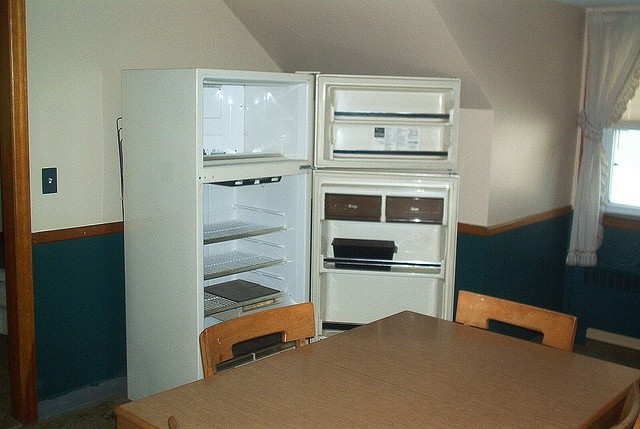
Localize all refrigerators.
[119,67,473,406]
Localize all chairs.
[454,290,578,351]
[199,302,316,379]
